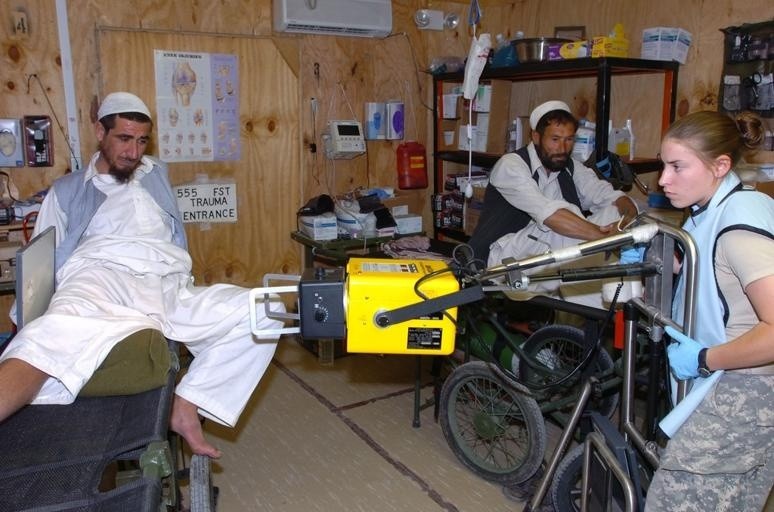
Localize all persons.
[0,90,289,459]
[641,109,774,511]
[466,99,642,303]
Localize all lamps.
[414,10,431,26]
[443,12,460,30]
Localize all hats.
[529,101,573,131]
[97,91,153,125]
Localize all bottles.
[624,118,635,161]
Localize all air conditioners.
[271,0,393,39]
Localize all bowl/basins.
[511,38,574,64]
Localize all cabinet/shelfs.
[432,55,679,340]
[717,19,774,200]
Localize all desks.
[290,229,473,427]
[0,221,36,291]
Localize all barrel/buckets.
[608,126,630,163]
[397,140,428,189]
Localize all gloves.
[664,325,705,381]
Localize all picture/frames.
[553,25,586,43]
